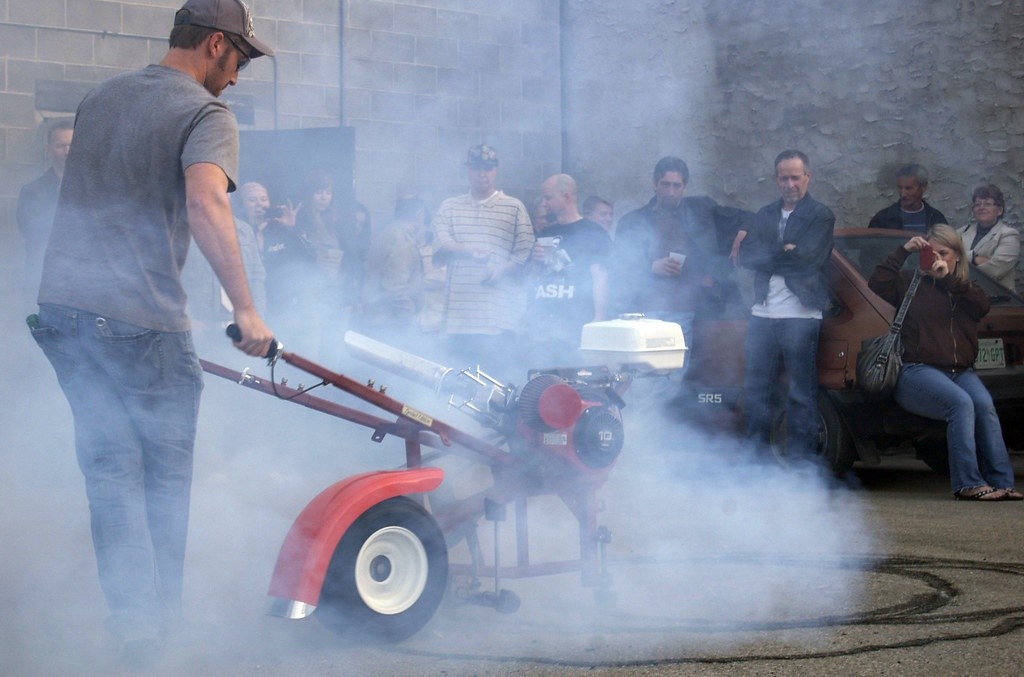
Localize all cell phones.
[920,246,933,271]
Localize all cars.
[680,228,1023,490]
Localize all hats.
[464,144,498,166]
[174,0,274,59]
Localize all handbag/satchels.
[855,333,905,400]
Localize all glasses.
[974,201,1002,207]
[470,164,493,171]
[211,31,251,71]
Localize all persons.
[583,196,614,232]
[522,174,614,323]
[182,145,535,351]
[957,184,1020,296]
[530,198,555,232]
[739,150,836,464]
[39,0,278,635]
[868,223,1024,502]
[16,121,73,263]
[859,164,948,278]
[614,156,757,414]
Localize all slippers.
[1005,488,1024,499]
[955,486,1008,500]
